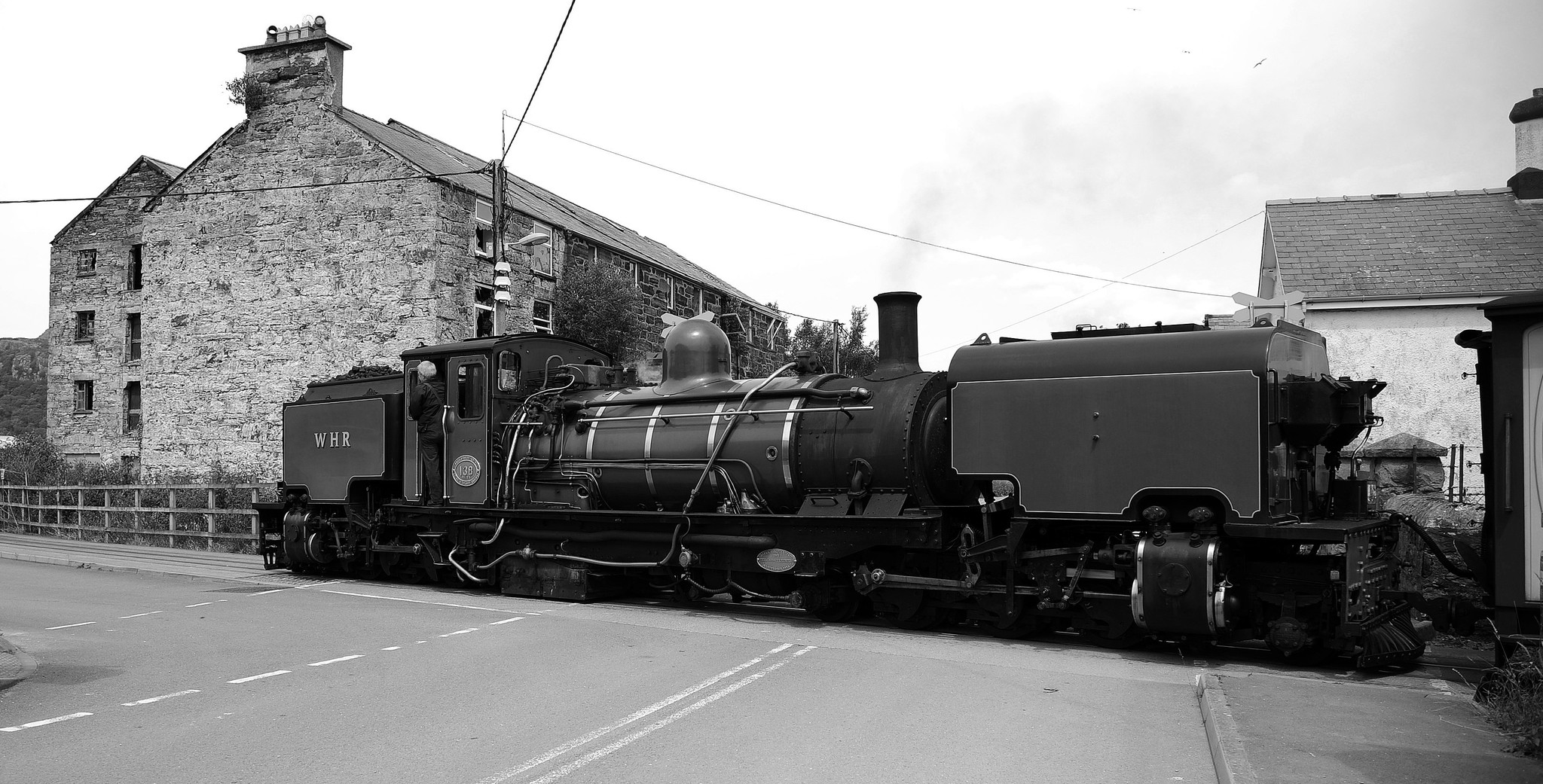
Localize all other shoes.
[426,499,444,507]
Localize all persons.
[409,360,446,507]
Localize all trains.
[248,291,1438,681]
